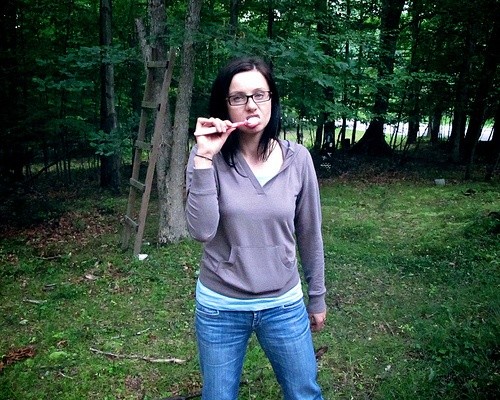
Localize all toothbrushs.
[194,117,261,136]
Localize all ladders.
[120,45,177,258]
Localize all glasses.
[225,91,272,106]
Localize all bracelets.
[196,154,213,163]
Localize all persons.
[185,58,327,400]
